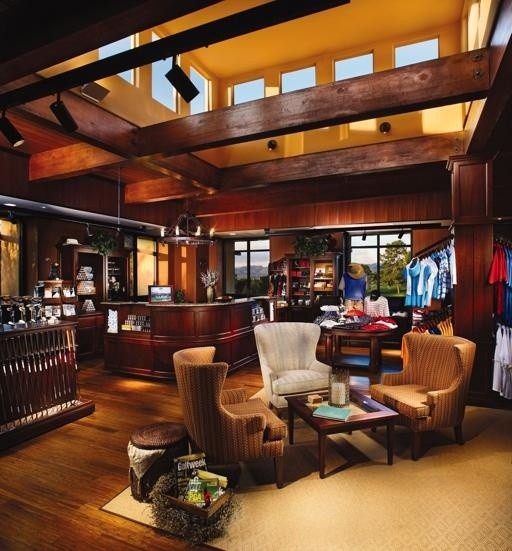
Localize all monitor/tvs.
[148,285,175,304]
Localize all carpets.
[100,388,512,551]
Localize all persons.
[362,290,390,318]
[337,270,369,313]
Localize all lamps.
[165,56,201,104]
[157,200,220,248]
[1,93,79,149]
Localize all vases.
[206,286,214,304]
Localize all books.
[312,405,352,422]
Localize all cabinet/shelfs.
[284,252,345,320]
[57,244,136,362]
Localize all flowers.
[200,269,222,288]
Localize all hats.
[346,262,364,279]
